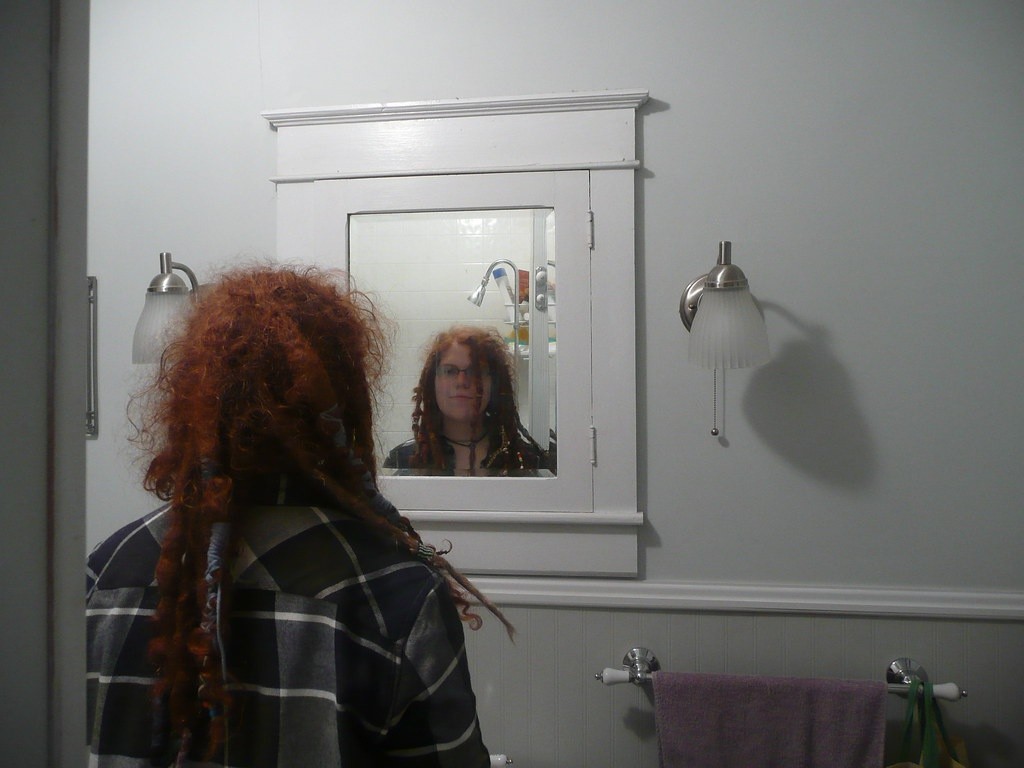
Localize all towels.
[650,670,887,768]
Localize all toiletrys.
[492,265,529,324]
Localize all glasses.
[436,363,489,379]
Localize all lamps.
[131,252,198,365]
[681,240,773,435]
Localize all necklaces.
[440,431,489,476]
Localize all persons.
[84,268,515,768]
[382,326,551,476]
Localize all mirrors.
[347,207,557,477]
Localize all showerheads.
[466,259,519,326]
